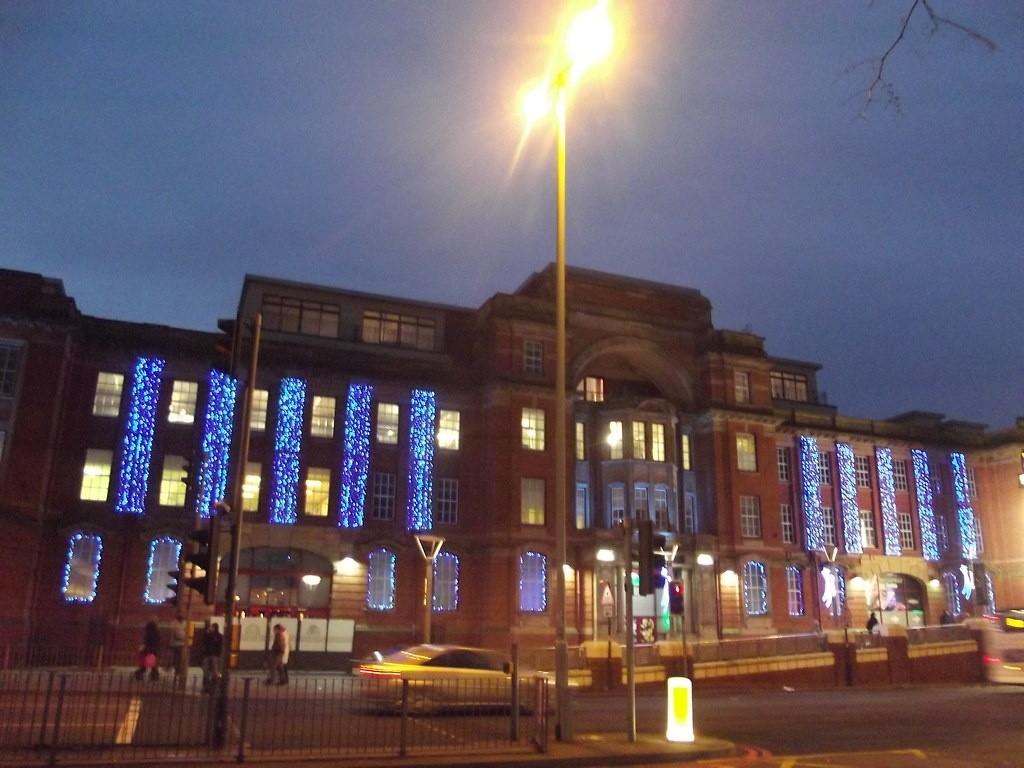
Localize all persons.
[866,613,878,634]
[134,622,161,680]
[169,613,185,676]
[264,624,289,685]
[940,609,970,624]
[200,624,224,693]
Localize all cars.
[351,643,578,716]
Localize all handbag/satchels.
[137,649,156,668]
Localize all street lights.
[516,8,625,741]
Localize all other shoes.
[263,679,274,684]
[275,680,287,686]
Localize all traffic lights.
[638,519,666,596]
[167,565,185,616]
[184,516,218,604]
[669,582,683,615]
[181,453,203,492]
[215,314,240,357]
